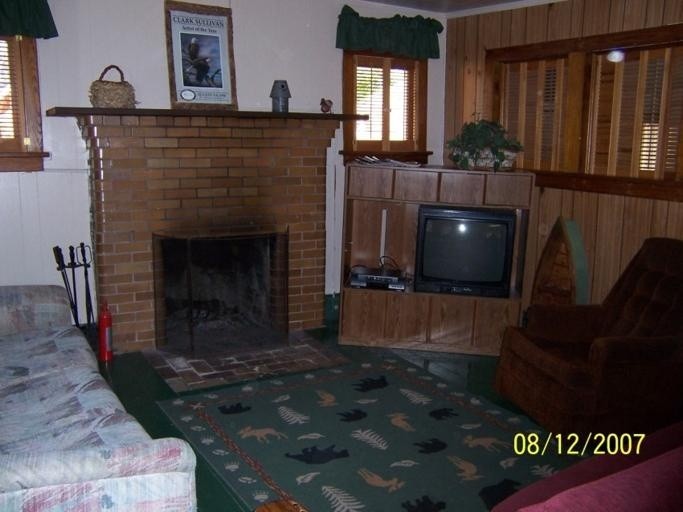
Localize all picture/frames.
[166,1,236,113]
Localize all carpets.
[157,353,579,511]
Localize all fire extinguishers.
[97,298,112,362]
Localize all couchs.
[0,284,197,512]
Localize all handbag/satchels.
[89,81,136,109]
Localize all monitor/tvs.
[413,204,516,299]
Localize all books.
[349,154,422,170]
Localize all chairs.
[491,236,681,450]
[487,422,683,512]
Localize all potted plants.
[446,119,524,171]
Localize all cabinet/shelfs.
[336,161,537,356]
